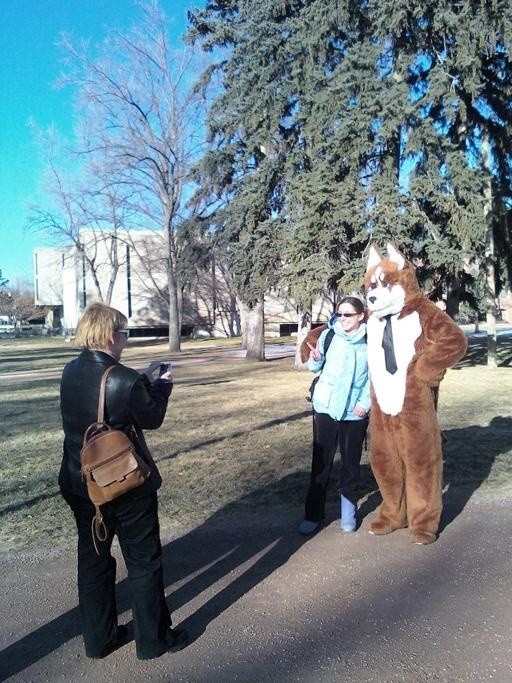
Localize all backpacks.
[78,362,153,527]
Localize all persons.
[298,295,371,537]
[57,301,190,660]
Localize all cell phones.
[159,364,169,377]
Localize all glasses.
[118,329,130,337]
[335,313,359,317]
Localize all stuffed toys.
[299,242,468,544]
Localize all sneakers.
[298,520,320,535]
